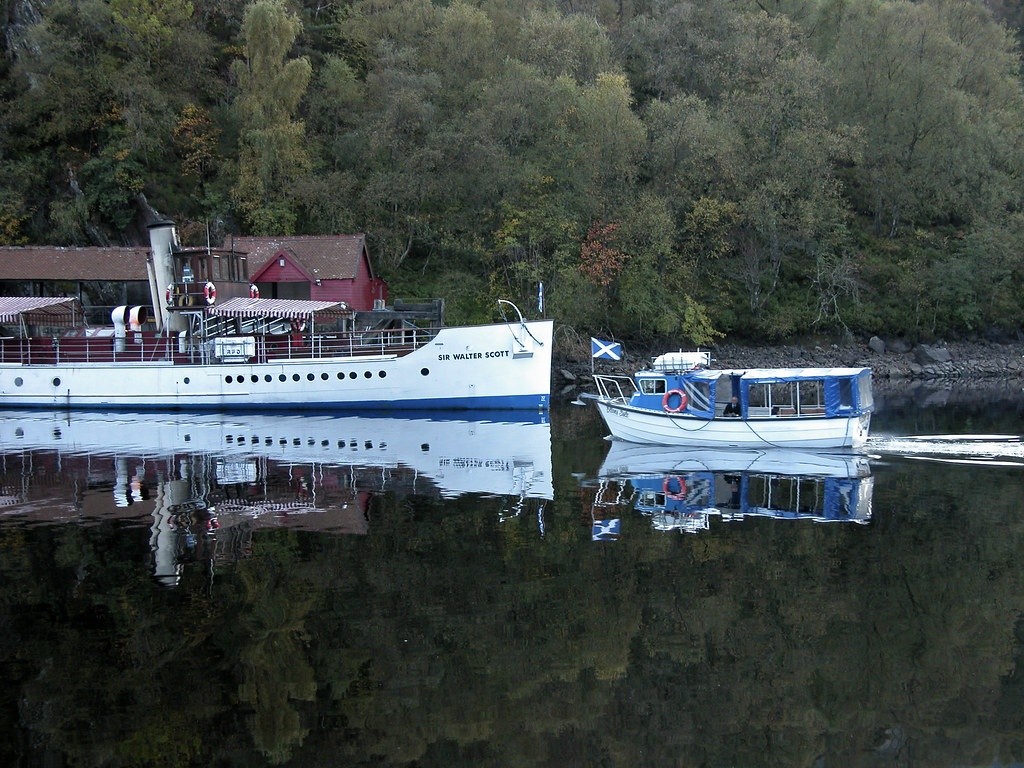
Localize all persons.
[723,397,742,417]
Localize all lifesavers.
[204,282,216,304]
[178,294,194,307]
[167,284,178,304]
[663,477,687,500]
[662,387,687,413]
[249,286,258,298]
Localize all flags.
[591,337,621,361]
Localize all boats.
[590,439,876,544]
[580,336,876,450]
[1,219,556,414]
[2,409,556,584]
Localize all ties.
[733,404,735,408]
